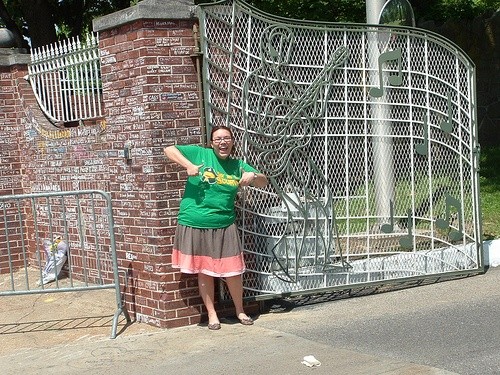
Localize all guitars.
[251,46,349,179]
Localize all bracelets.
[251,172,257,186]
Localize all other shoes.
[237,316,253,325]
[208,321,220,330]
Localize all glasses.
[211,136,234,143]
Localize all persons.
[162,125,269,329]
